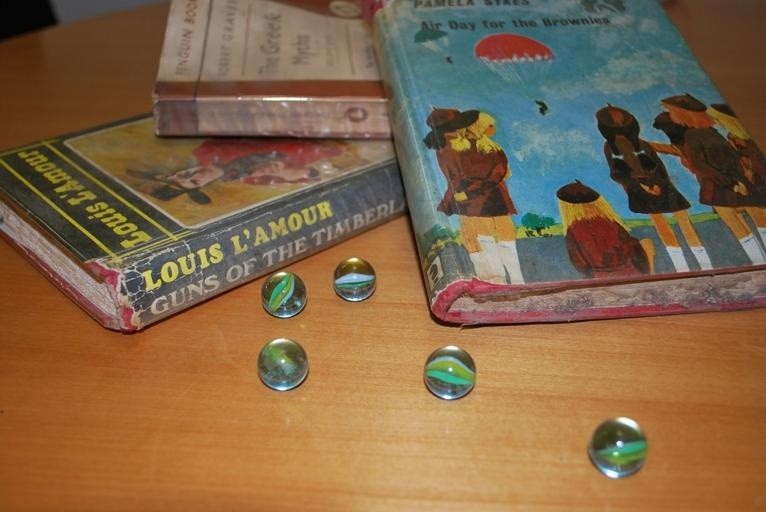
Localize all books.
[364,0,765,327]
[151,1,390,144]
[0,113,408,332]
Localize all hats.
[125,166,213,205]
[662,92,707,112]
[595,103,634,129]
[712,104,736,118]
[426,108,480,132]
[554,180,600,204]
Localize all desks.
[1,2,766,511]
